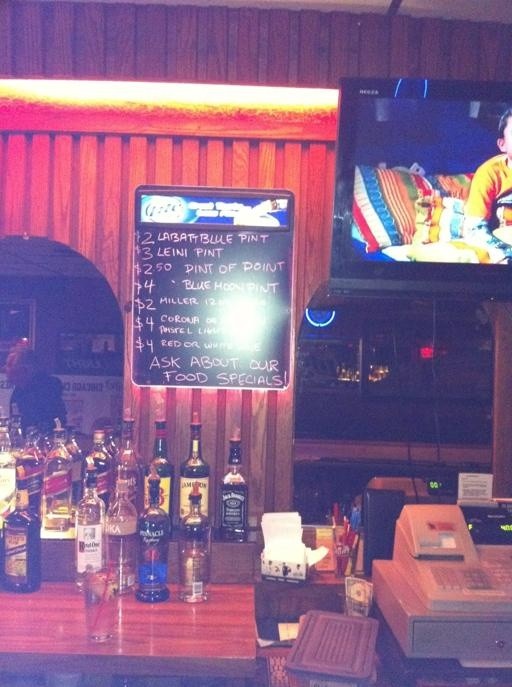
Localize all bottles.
[0,414,176,602]
[177,482,209,603]
[177,423,209,526]
[218,429,248,544]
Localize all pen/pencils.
[331,501,365,575]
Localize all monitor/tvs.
[327,75,512,301]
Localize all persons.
[9,350,67,442]
[460,107,512,265]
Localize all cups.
[83,569,115,642]
[332,527,357,580]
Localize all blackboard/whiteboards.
[131,185,295,390]
